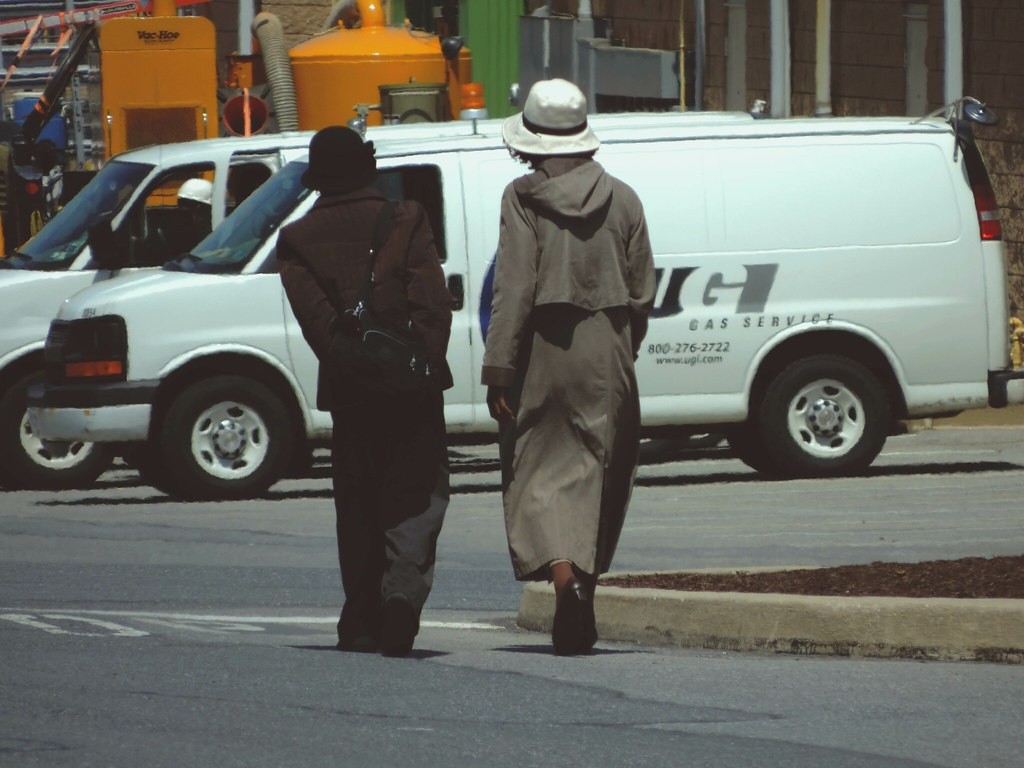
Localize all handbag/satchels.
[354,200,432,414]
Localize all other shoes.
[337,618,372,647]
[552,577,598,650]
[381,601,416,653]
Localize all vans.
[0,109,758,497]
[24,103,1014,480]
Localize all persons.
[278,125,453,657]
[481,79,657,654]
[157,179,212,260]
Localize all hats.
[299,125,376,194]
[502,77,600,154]
[177,177,214,205]
